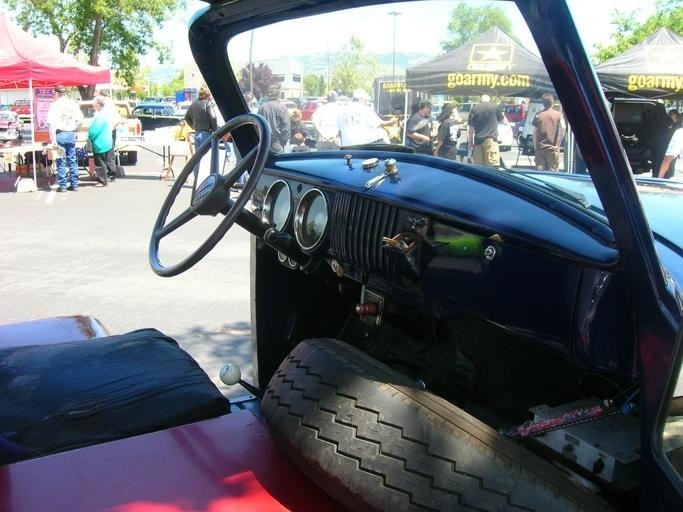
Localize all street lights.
[388,12,403,81]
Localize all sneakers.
[67,186,78,191]
[57,188,65,192]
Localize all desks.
[0,140,225,190]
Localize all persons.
[231,87,254,183]
[432,102,461,159]
[87,95,116,187]
[46,84,84,192]
[667,109,681,126]
[258,85,399,154]
[466,94,505,168]
[405,98,435,154]
[529,93,567,172]
[658,127,683,183]
[93,88,121,146]
[184,85,218,171]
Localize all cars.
[457,102,515,153]
[133,95,192,125]
[499,104,526,123]
[258,96,269,107]
[298,100,322,125]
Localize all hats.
[54,85,65,93]
[419,99,432,107]
[354,89,370,100]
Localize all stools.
[0,325,228,469]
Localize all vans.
[608,98,664,173]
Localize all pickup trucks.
[77,99,144,163]
